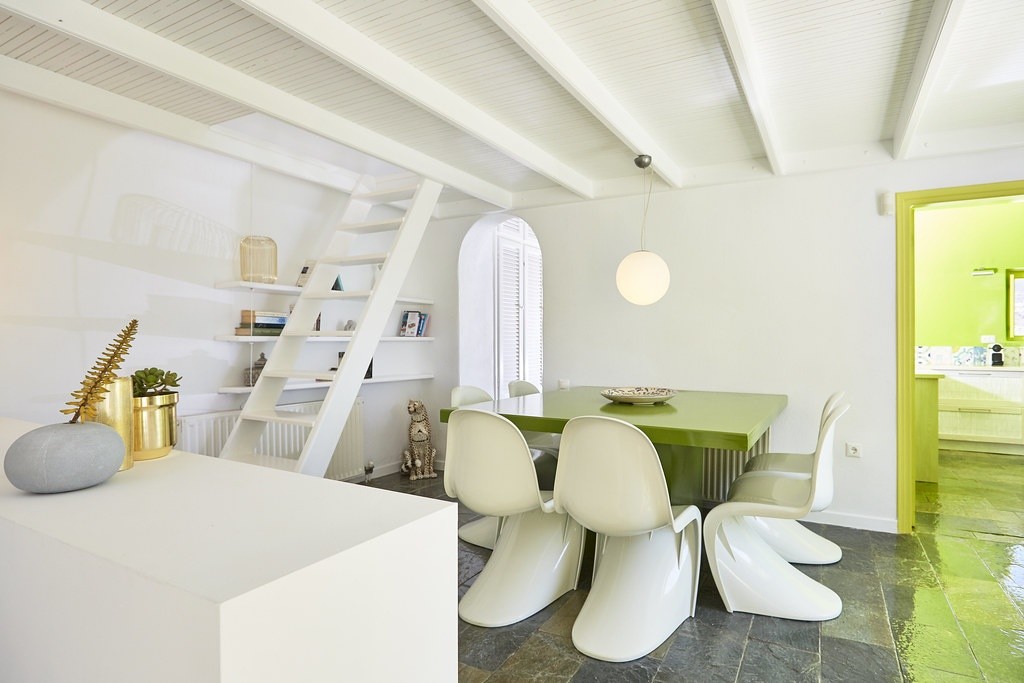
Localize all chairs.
[553,415,702,663]
[451,385,548,546]
[706,403,857,623]
[737,380,848,565]
[506,380,566,462]
[443,407,583,627]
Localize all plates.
[600,385,679,405]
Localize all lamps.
[617,146,675,307]
[172,399,369,481]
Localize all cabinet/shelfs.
[217,281,434,397]
[0,411,458,683]
[922,365,1024,456]
[914,365,945,483]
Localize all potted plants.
[130,366,181,461]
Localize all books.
[297,267,312,287]
[235,310,289,335]
[400,311,429,337]
[332,275,343,290]
[313,315,320,331]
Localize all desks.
[441,385,790,559]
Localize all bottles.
[240,235,278,284]
